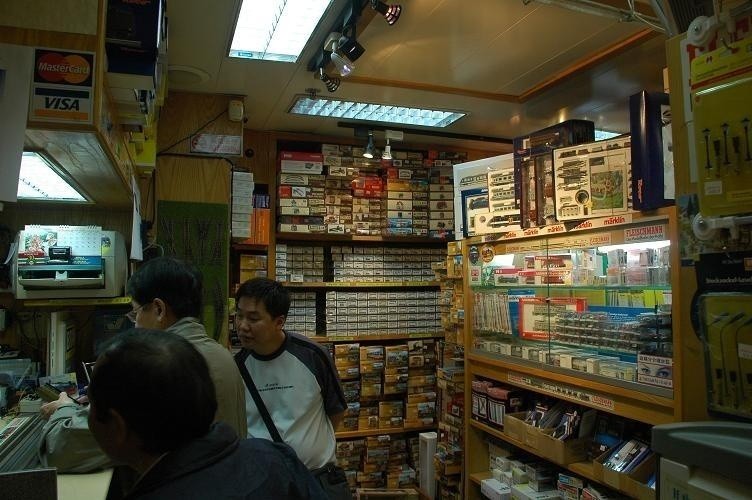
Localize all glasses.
[124,304,154,323]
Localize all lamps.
[307,0,401,92]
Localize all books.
[0,414,46,476]
[475,290,535,338]
[106,17,167,178]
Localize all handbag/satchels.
[307,462,351,500]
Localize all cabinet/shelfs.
[465,205,683,500]
[269,132,464,500]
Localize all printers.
[17,229,128,299]
